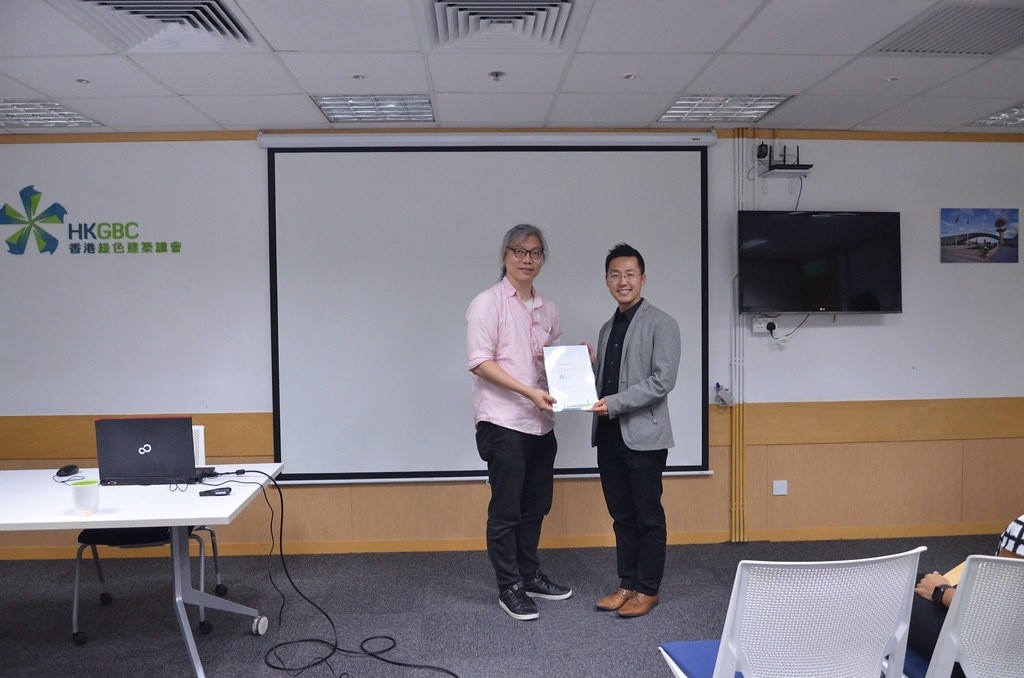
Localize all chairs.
[903,555,1024,678]
[71,425,226,646]
[659,543,928,678]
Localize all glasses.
[507,246,543,260]
[607,272,642,282]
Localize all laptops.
[95,417,215,486]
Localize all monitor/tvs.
[737,210,903,316]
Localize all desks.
[0,461,283,678]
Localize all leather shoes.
[596,587,634,610]
[618,592,658,617]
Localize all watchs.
[932,585,951,603]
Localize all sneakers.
[498,580,539,620]
[521,569,572,600]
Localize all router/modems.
[768,145,813,170]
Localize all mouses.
[56,464,79,477]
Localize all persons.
[579,243,681,617]
[907,514,1024,678]
[467,223,573,620]
